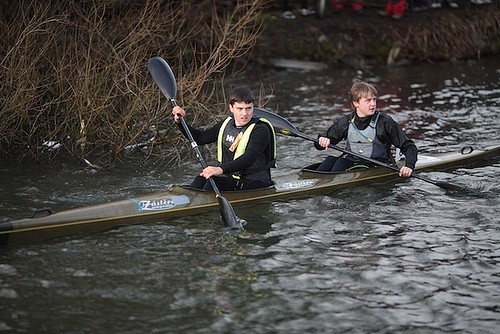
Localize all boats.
[0,141,500,246]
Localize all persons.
[172,87,277,191]
[314,82,418,177]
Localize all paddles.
[252,108,475,191]
[147,56,238,226]
